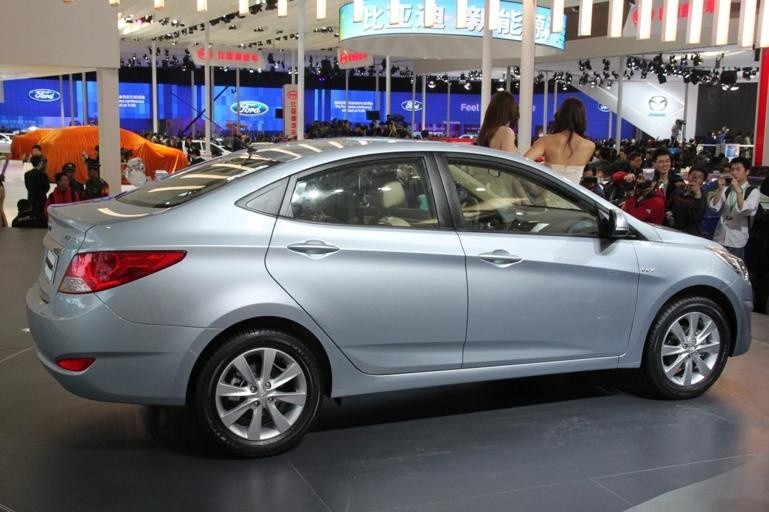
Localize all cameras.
[725,176,734,187]
[624,181,635,191]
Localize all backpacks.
[725,185,769,252]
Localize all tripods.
[671,130,682,148]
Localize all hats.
[63,159,100,172]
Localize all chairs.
[376,181,412,227]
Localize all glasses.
[630,159,704,179]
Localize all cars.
[24,135,755,460]
[457,134,479,146]
[0,132,19,155]
[158,137,276,174]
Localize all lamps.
[109,0,769,94]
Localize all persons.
[476,91,761,261]
[307,114,412,139]
[0,145,109,227]
[144,129,281,166]
